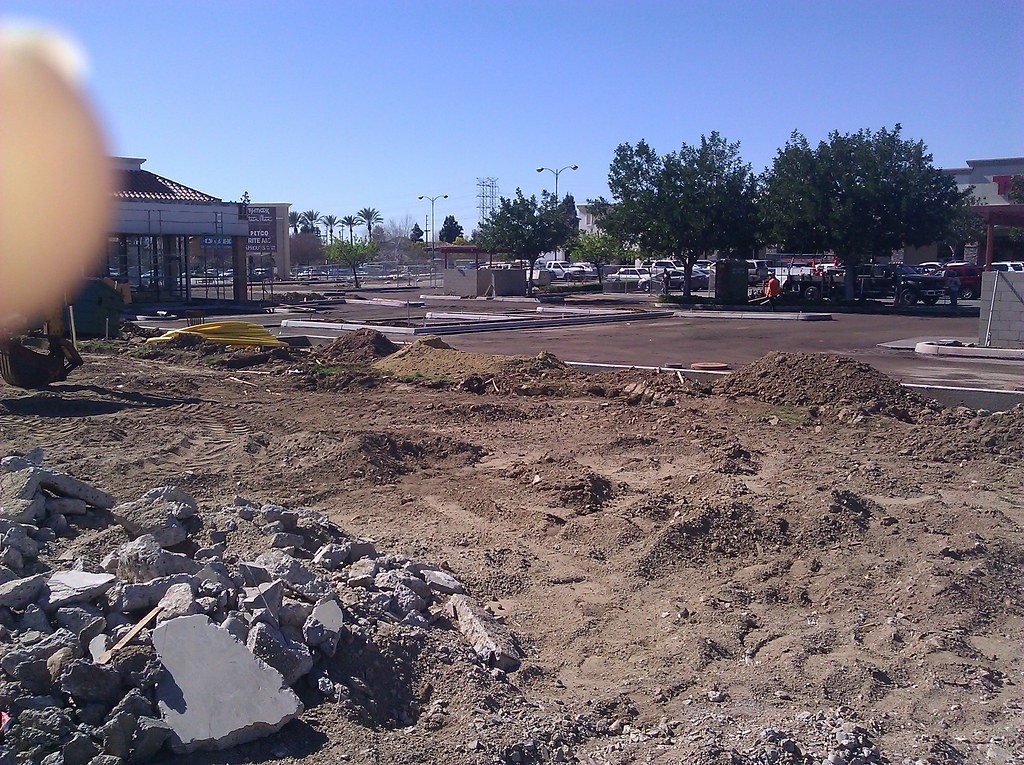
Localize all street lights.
[417,193,449,265]
[535,164,579,261]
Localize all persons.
[660,268,672,294]
[947,271,961,305]
[766,272,780,311]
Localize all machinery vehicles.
[0,275,132,390]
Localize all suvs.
[855,262,947,308]
[928,263,983,300]
[982,261,1024,271]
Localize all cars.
[605,258,847,304]
[110,265,323,280]
[463,261,597,282]
